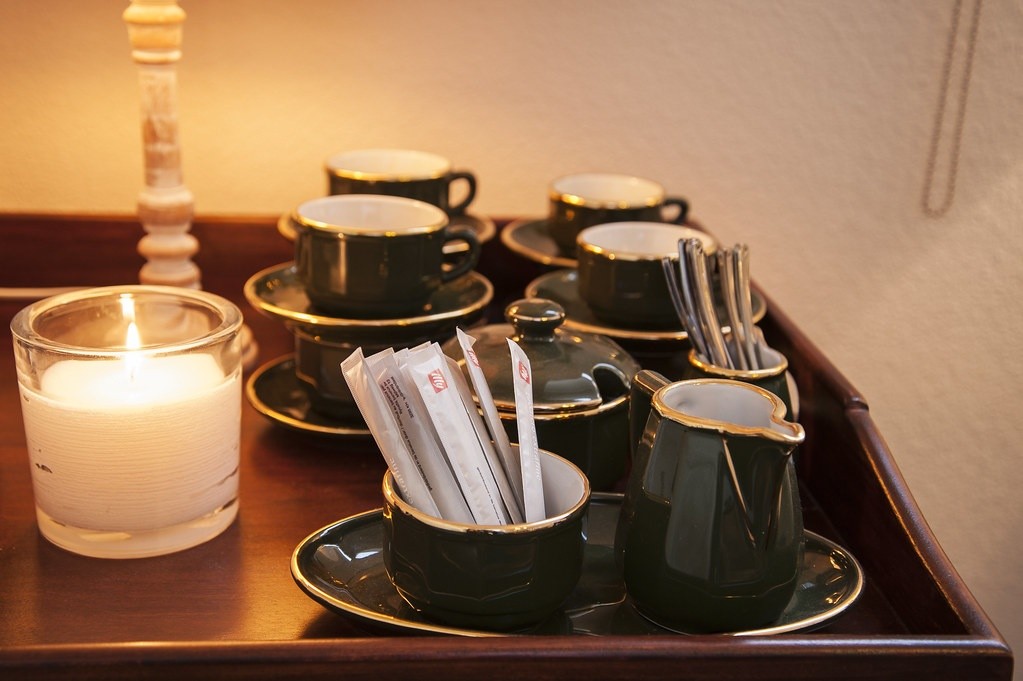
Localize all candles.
[42,320,227,404]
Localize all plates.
[287,496,863,638]
[278,208,496,255]
[243,260,494,336]
[245,348,368,436]
[528,268,767,345]
[500,213,714,267]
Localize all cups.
[294,192,478,315]
[686,341,794,423]
[9,284,244,558]
[324,148,478,224]
[291,328,364,406]
[381,442,592,628]
[548,172,690,257]
[575,222,721,325]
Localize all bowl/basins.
[437,296,644,495]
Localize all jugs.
[613,370,808,633]
